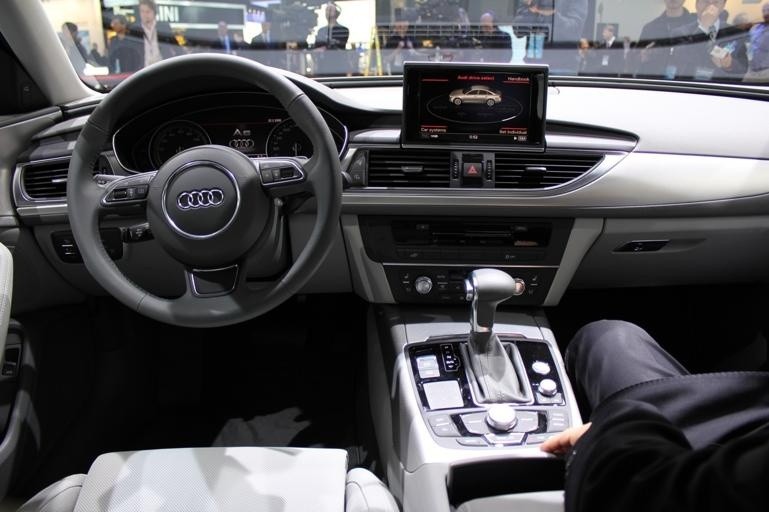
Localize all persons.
[210,21,239,57]
[539,319,769,512]
[59,0,186,74]
[250,20,280,68]
[384,18,419,74]
[480,0,769,84]
[314,2,349,76]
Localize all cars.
[449,85,502,107]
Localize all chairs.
[9,446,401,512]
